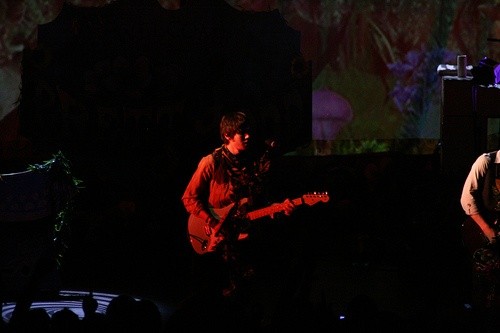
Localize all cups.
[457,55,467,77]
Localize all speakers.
[441,76,488,191]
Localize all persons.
[179,110,300,300]
[458,146,500,304]
[0,258,499,333]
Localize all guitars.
[462,209,500,252]
[187,188,332,254]
